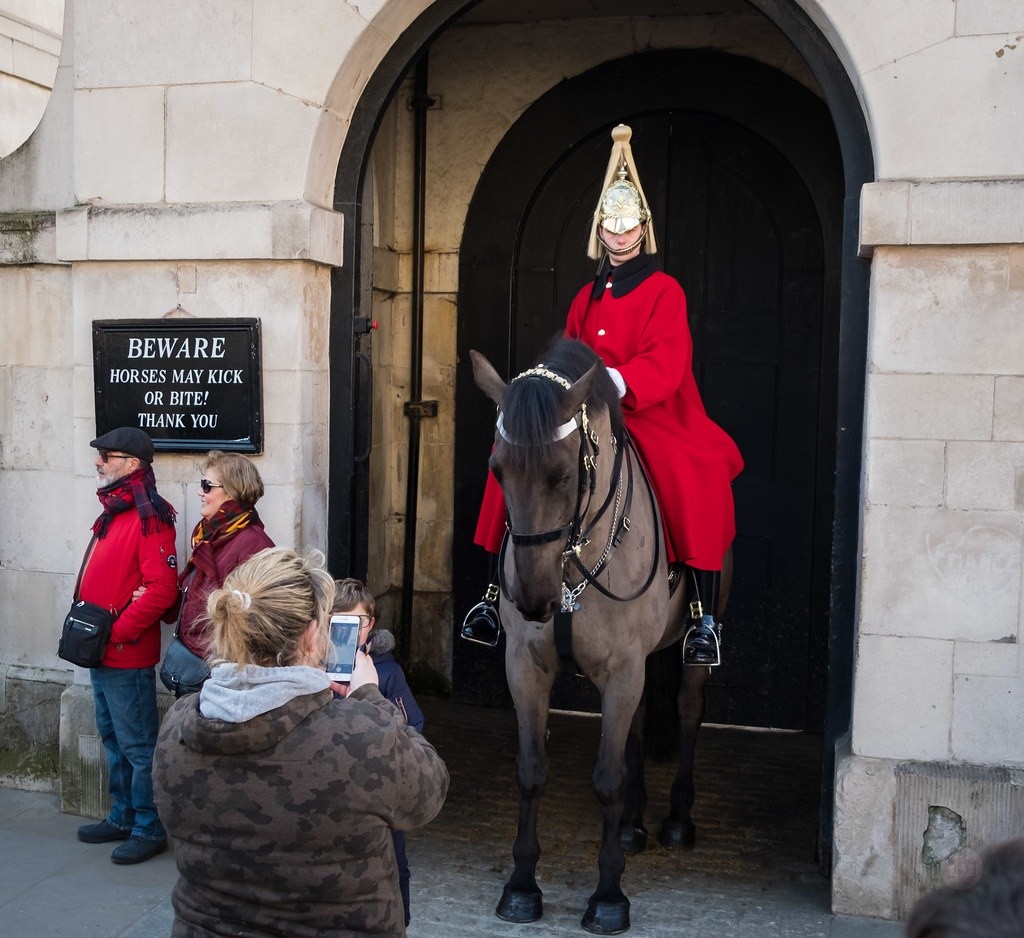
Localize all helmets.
[586,124,657,259]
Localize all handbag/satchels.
[160,639,210,698]
[59,600,113,668]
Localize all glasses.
[357,612,372,629]
[200,479,223,494]
[98,450,135,462]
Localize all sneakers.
[78,819,130,844]
[111,834,169,865]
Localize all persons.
[462,124,743,664]
[903,837,1024,938]
[73,427,179,865]
[329,578,424,928]
[131,451,276,699]
[151,546,450,938]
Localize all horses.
[465,333,735,931]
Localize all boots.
[684,565,720,664]
[462,553,502,645]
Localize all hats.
[89,427,154,463]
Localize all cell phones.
[323,616,362,683]
